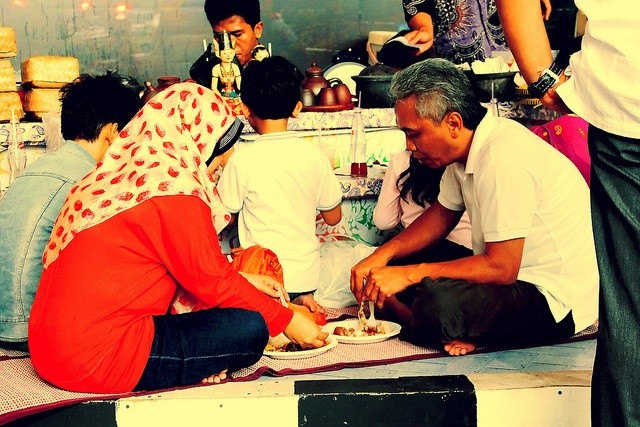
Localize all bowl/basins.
[473,71,520,102]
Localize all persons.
[529,33,591,189]
[250,42,272,61]
[29,81,330,393]
[495,0,640,426]
[0,68,139,352]
[373,149,473,249]
[204,0,264,69]
[216,55,343,317]
[402,0,552,65]
[211,29,242,105]
[350,57,600,355]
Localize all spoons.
[358,278,369,330]
[366,301,379,334]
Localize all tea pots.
[144,77,197,110]
[299,63,341,103]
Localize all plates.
[323,61,368,101]
[323,318,401,345]
[263,332,338,360]
[301,106,355,112]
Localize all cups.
[320,88,336,106]
[42,114,62,155]
[334,84,351,105]
[302,89,316,107]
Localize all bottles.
[6,119,27,187]
[352,108,367,178]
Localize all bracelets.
[527,61,563,99]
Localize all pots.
[351,62,402,108]
